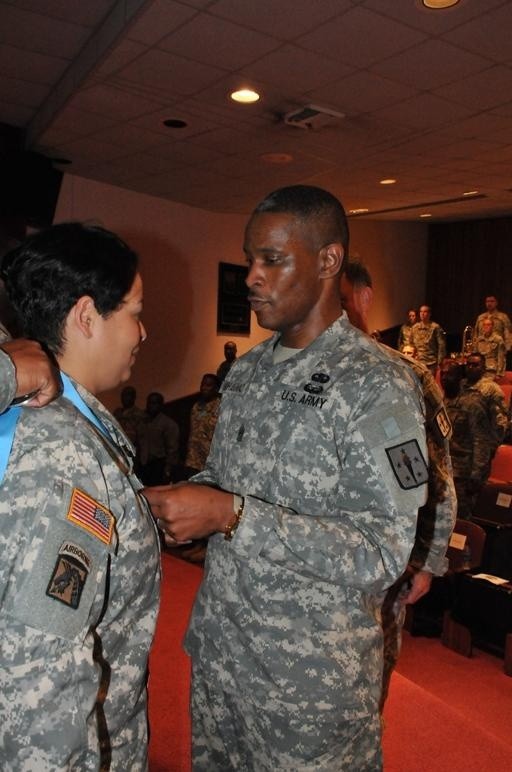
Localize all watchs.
[225,496,244,543]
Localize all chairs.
[406,355,512,677]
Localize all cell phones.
[11,390,40,405]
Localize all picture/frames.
[217,262,249,337]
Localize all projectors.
[281,103,345,132]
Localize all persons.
[113,294,511,623]
[138,185,432,770]
[334,261,458,737]
[0,221,164,771]
[0,305,60,413]
